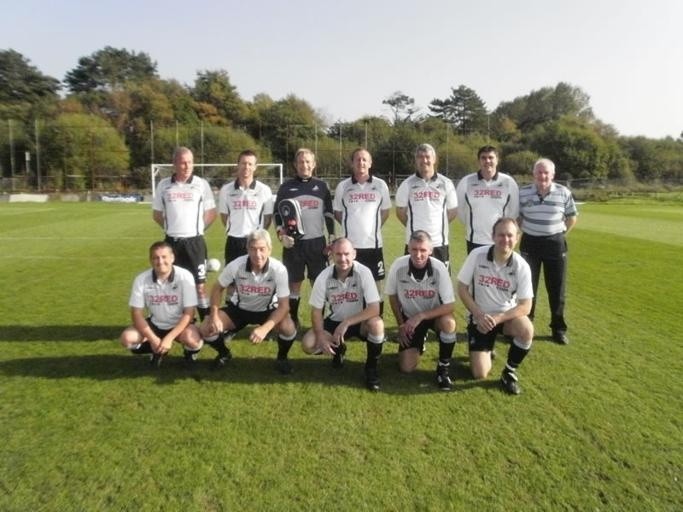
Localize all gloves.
[279,233,294,249]
[323,235,335,264]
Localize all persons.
[504,158,578,345]
[333,148,392,319]
[302,237,385,390]
[120,241,203,368]
[457,217,534,394]
[385,230,457,391]
[273,148,335,326]
[219,151,275,342]
[152,145,218,320]
[200,230,298,373]
[396,143,458,276]
[456,146,520,254]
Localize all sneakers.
[501,374,519,396]
[433,372,453,390]
[277,359,291,372]
[212,353,232,367]
[182,356,197,367]
[554,332,568,344]
[150,353,163,366]
[332,343,347,366]
[367,372,382,390]
